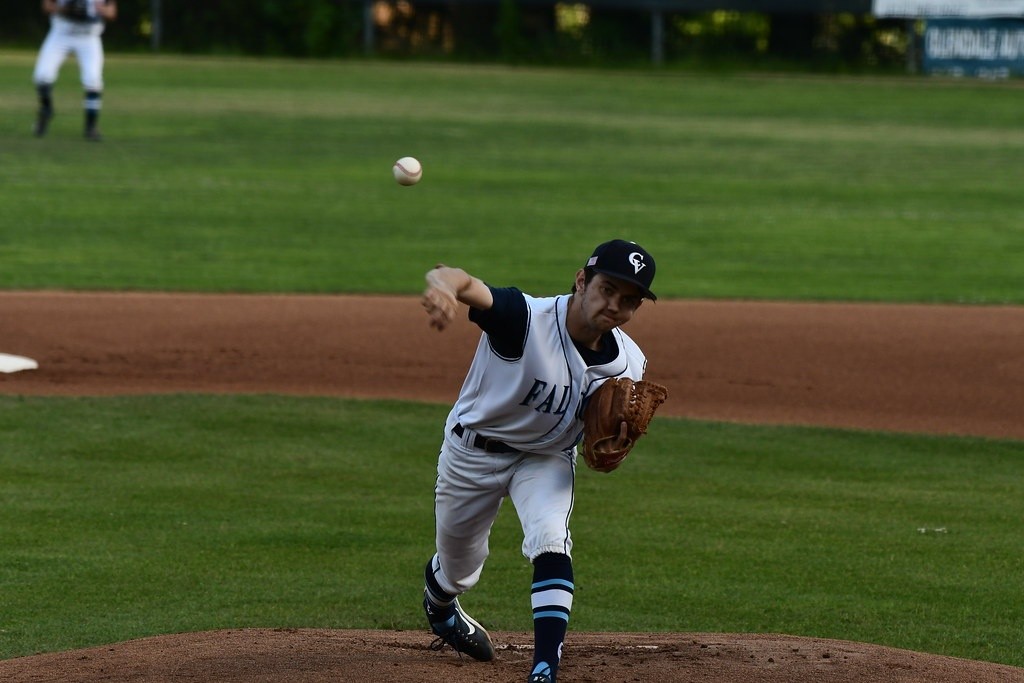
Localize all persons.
[31,0,118,140]
[421,240,669,683]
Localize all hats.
[586,239,658,300]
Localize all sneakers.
[423,587,495,662]
[527,666,556,683]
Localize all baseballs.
[393,154,423,186]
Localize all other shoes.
[85,130,100,141]
[33,111,48,137]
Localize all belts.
[452,423,522,453]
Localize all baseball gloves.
[582,373,671,474]
